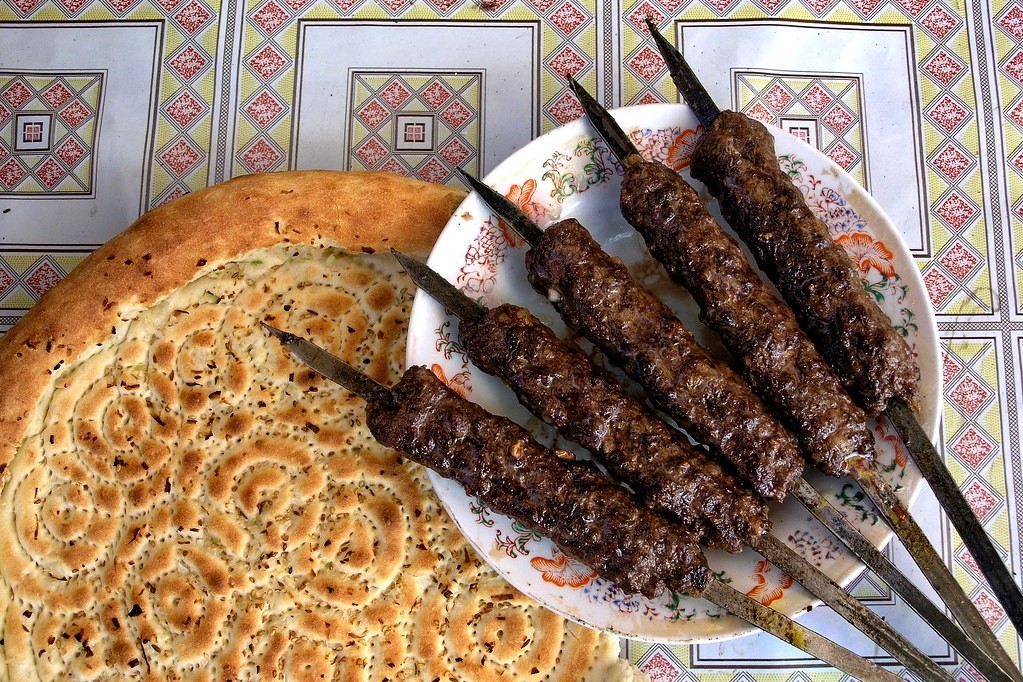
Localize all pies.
[0,169,647,682]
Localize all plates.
[403,99,946,643]
